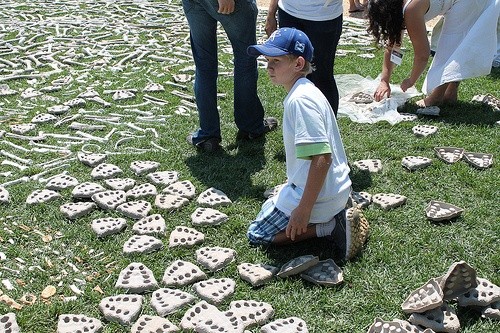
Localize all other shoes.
[347,202,370,248]
[186,133,221,146]
[237,134,265,145]
[349,8,364,12]
[397,100,430,114]
[330,207,362,262]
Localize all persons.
[367,0,500,112]
[182,0,278,151]
[247,28,371,261]
[263,0,357,118]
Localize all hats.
[247,27,314,63]
